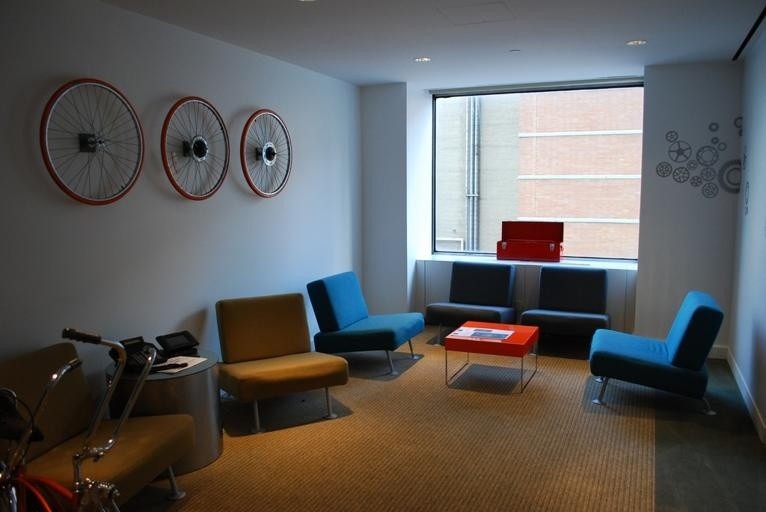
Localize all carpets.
[119,325,657,512]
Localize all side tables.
[105,349,223,478]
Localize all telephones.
[109,336,167,373]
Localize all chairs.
[426,261,517,346]
[0,343,197,512]
[589,290,724,416]
[215,293,349,431]
[306,271,426,377]
[520,265,611,361]
